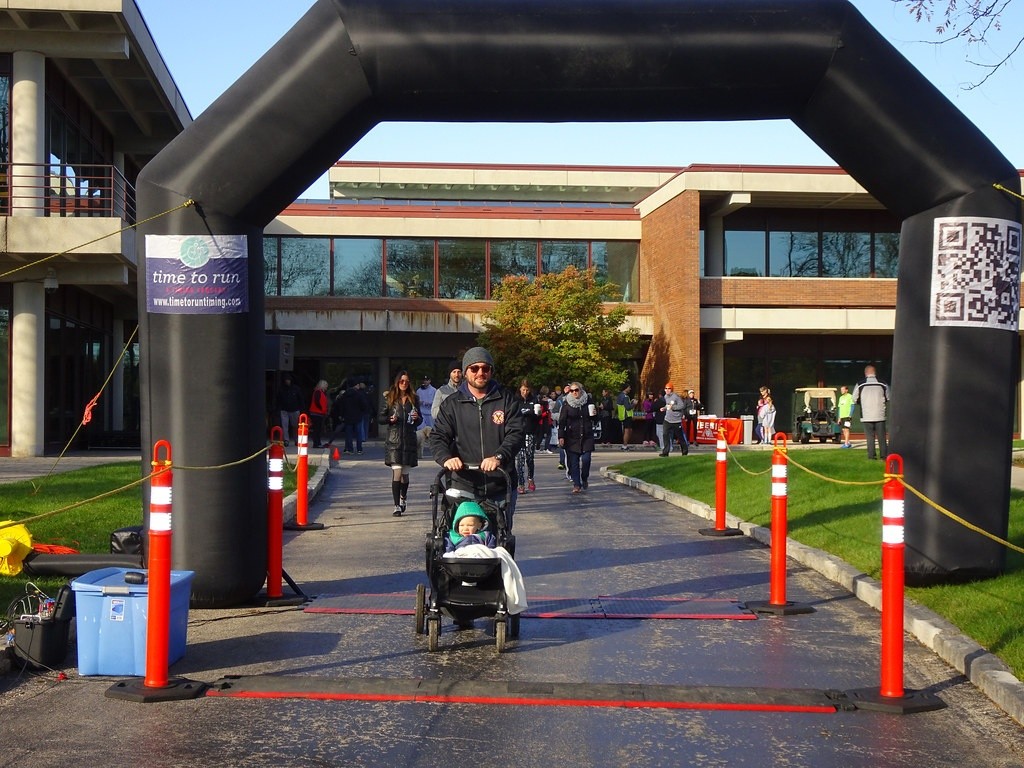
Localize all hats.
[462,347,495,377]
[621,381,630,390]
[424,375,431,381]
[688,390,694,394]
[555,386,561,391]
[646,391,655,395]
[665,382,673,392]
[448,361,462,379]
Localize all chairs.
[816,410,828,422]
[0,173,9,212]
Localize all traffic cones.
[333,448,340,459]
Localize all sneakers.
[358,446,364,454]
[543,449,553,454]
[527,477,535,491]
[519,484,524,494]
[535,450,543,454]
[399,491,407,511]
[583,480,587,489]
[841,443,851,449]
[558,463,566,469]
[572,484,581,493]
[342,449,354,455]
[392,504,401,517]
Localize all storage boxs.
[71,567,195,678]
[14,585,75,671]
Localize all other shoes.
[643,441,655,446]
[621,445,633,452]
[659,452,668,457]
[452,616,474,629]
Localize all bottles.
[407,407,416,424]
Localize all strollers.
[413,463,521,652]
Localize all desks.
[633,416,646,420]
[682,418,743,445]
[591,418,657,444]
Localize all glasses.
[570,389,579,393]
[399,380,409,385]
[468,365,492,373]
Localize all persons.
[378,370,424,517]
[444,502,496,553]
[279,362,465,454]
[837,385,854,449]
[755,385,777,444]
[430,347,526,626]
[852,365,890,460]
[515,380,701,493]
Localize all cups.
[588,403,595,416]
[534,404,540,414]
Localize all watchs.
[495,453,505,461]
